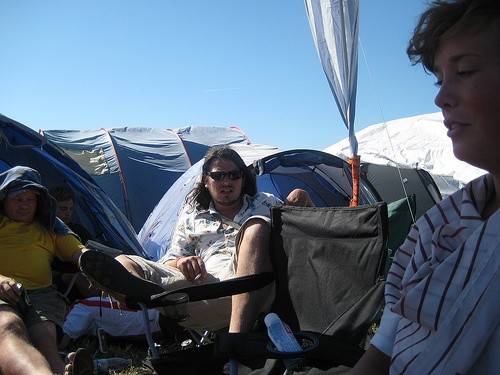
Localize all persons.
[344,0,500,375]
[78,147,316,355]
[0,165,110,375]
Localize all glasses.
[207,171,242,181]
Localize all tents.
[0,113,466,351]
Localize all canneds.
[180,339,195,349]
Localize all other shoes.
[79,250,165,308]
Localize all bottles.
[264,312,304,368]
[93,357,132,375]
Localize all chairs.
[141,194,418,375]
[51,258,84,294]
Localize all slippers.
[63,348,93,375]
